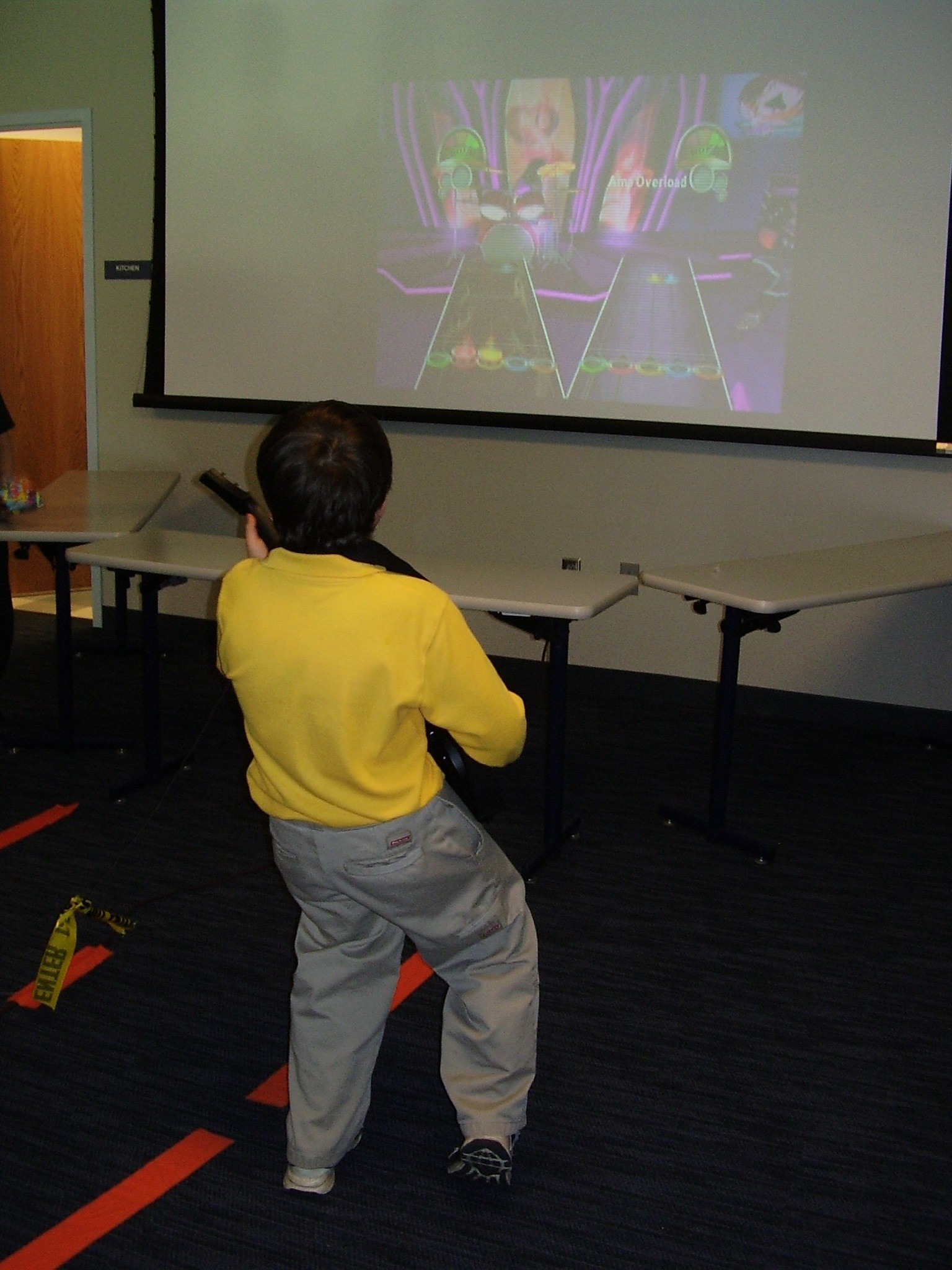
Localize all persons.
[0,393,15,727]
[215,399,541,1195]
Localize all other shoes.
[284,1166,338,1194]
[447,1137,516,1186]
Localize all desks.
[65,528,638,886]
[638,529,952,865]
[0,469,180,756]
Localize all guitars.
[192,476,469,785]
